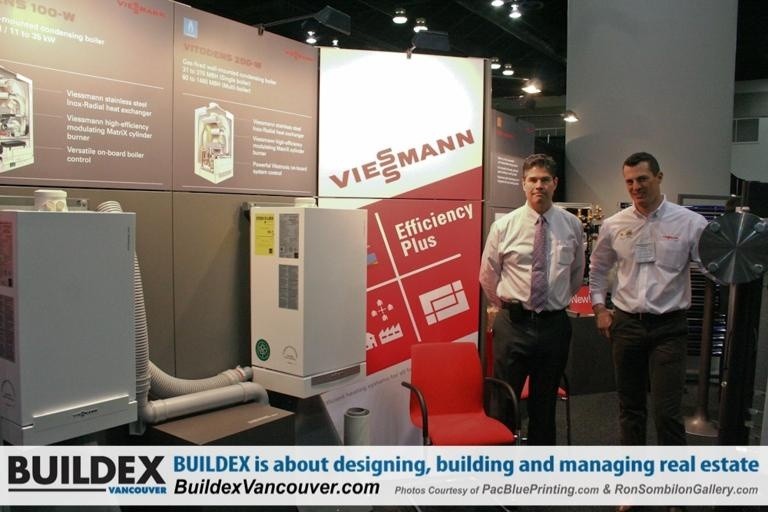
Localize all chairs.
[402,341,521,446]
[522,374,572,446]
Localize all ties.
[531,217,548,314]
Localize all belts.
[624,313,661,321]
[524,310,557,319]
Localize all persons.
[588,152,730,512]
[477,153,586,444]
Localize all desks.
[151,402,294,443]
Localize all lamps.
[392,1,429,35]
[489,51,514,76]
[520,77,542,94]
[491,0,521,19]
[306,26,339,46]
[562,112,579,122]
